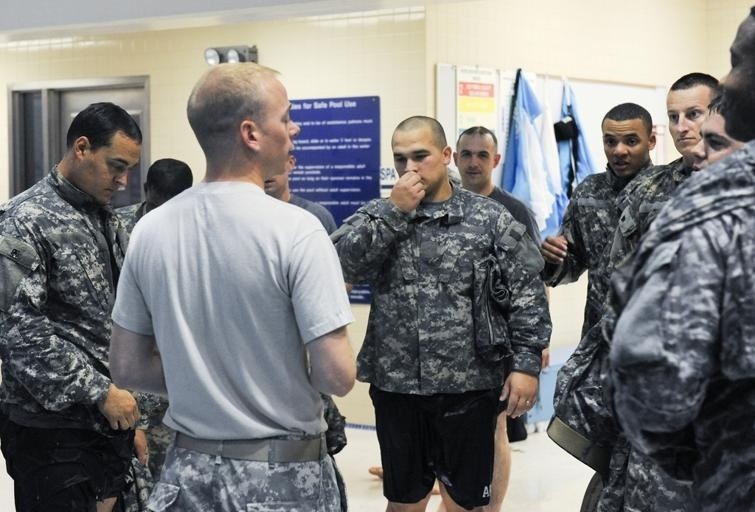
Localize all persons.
[369,467,440,495]
[112,158,193,512]
[264,150,352,297]
[329,116,553,512]
[108,62,357,512]
[0,102,149,512]
[540,4,755,510]
[436,126,549,512]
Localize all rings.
[526,400,532,406]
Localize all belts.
[548,416,610,472]
[175,433,320,463]
